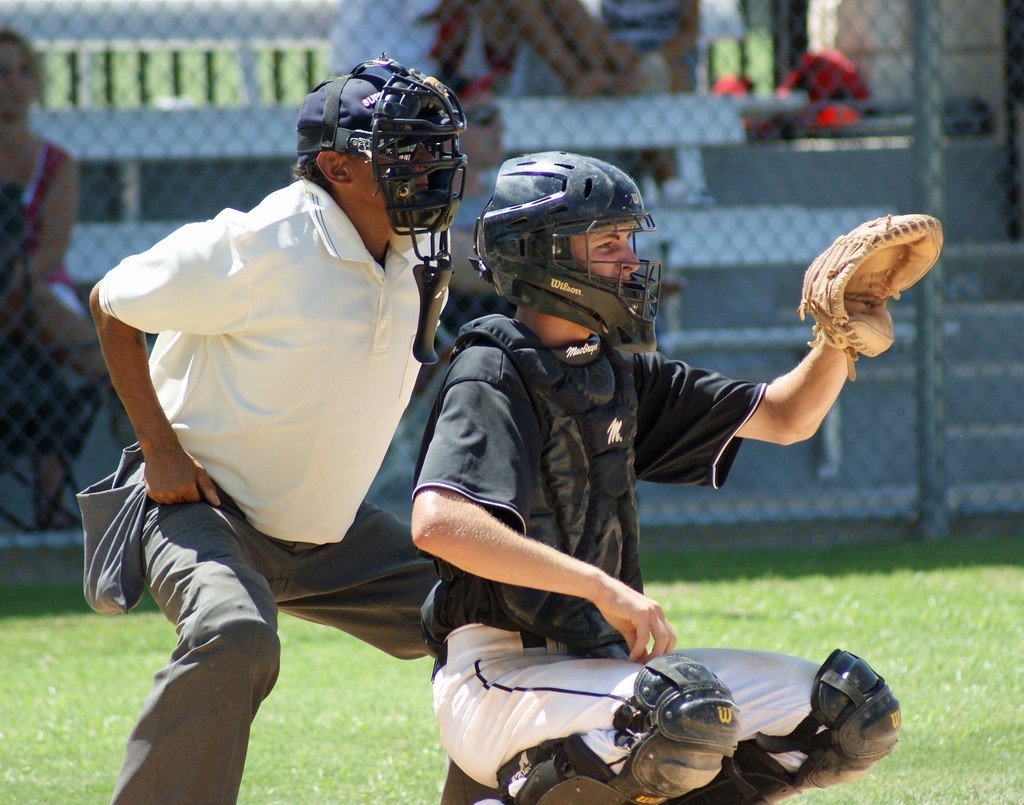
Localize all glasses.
[466,106,501,125]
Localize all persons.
[0,28,107,529]
[326,0,701,204]
[90,65,469,805]
[412,146,943,805]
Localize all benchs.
[28,90,898,485]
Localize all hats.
[296,73,458,165]
[431,63,505,102]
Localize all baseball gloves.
[795,212,944,385]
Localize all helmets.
[468,149,663,353]
